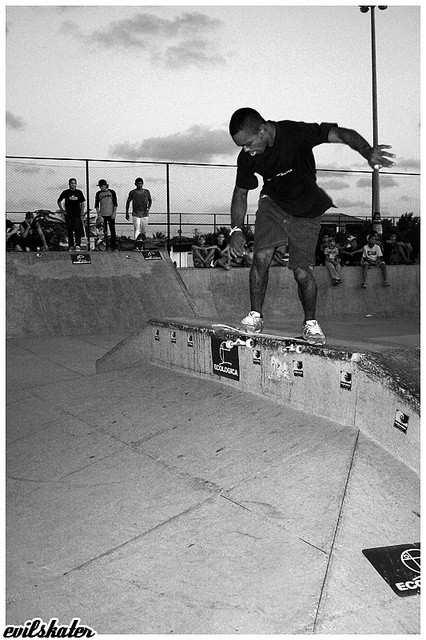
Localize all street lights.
[359,6,388,220]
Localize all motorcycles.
[30,209,91,238]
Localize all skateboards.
[132,233,145,252]
[212,324,308,354]
[94,217,106,251]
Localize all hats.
[96,179,107,186]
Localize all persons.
[228,108,397,346]
[57,178,85,252]
[324,237,344,286]
[125,178,152,252]
[211,233,233,270]
[191,235,218,268]
[370,231,385,261]
[386,232,415,265]
[94,179,119,251]
[245,241,256,267]
[5,219,23,252]
[316,233,333,266]
[360,234,391,288]
[19,212,49,252]
[341,235,364,266]
[270,245,288,267]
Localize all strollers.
[83,212,108,252]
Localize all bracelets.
[228,226,242,236]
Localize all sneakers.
[68,246,74,251]
[302,319,326,345]
[75,246,81,251]
[240,311,264,332]
